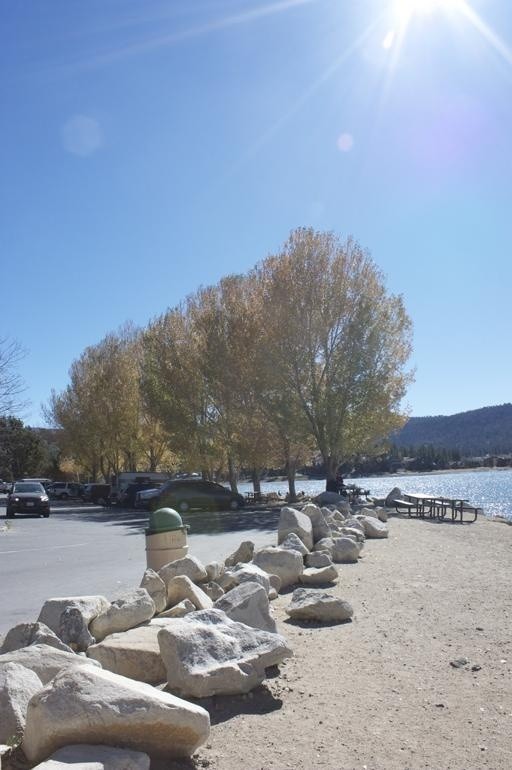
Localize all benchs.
[393,499,482,523]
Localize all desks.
[404,493,439,520]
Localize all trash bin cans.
[143,507,190,572]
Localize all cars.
[4,482,52,517]
[135,478,244,514]
[22,472,168,509]
